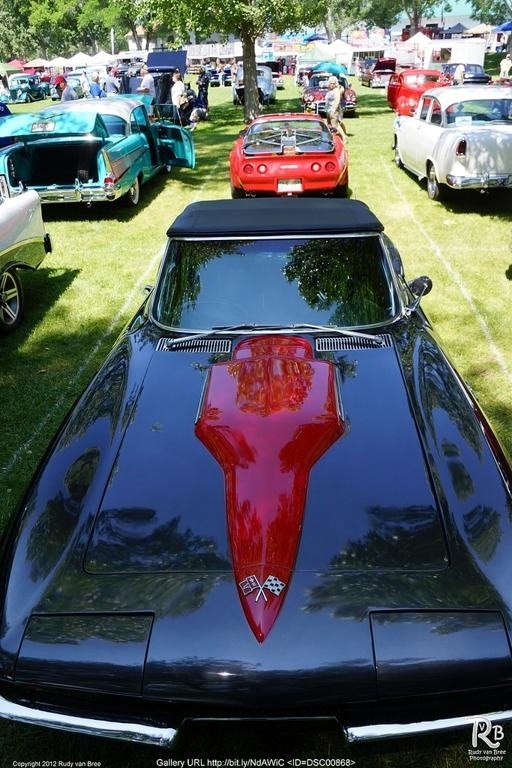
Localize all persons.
[0,53,511,144]
[496,33,507,55]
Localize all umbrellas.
[464,23,496,34]
[491,20,512,32]
[440,23,468,34]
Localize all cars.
[0,174,54,344]
[1,191,512,767]
[1,24,512,222]
